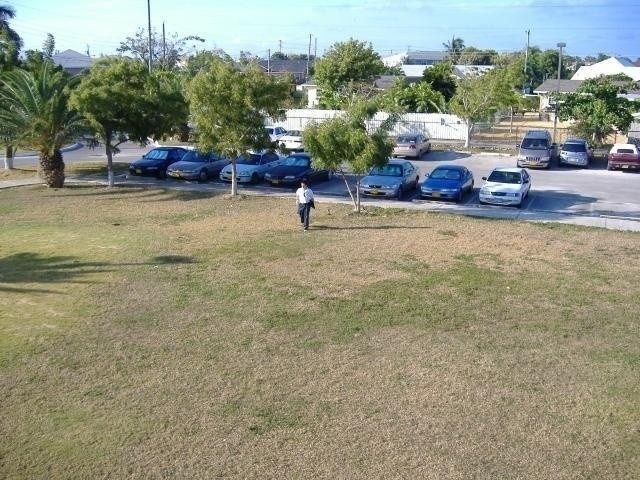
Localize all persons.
[295,178,315,232]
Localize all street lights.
[553,42,567,141]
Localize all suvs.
[515,129,556,167]
[559,139,595,167]
[607,144,640,172]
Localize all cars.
[166,150,231,182]
[420,165,474,202]
[255,126,286,148]
[478,167,531,208]
[130,147,187,179]
[393,134,432,158]
[356,161,420,199]
[264,153,336,186]
[219,151,286,183]
[279,128,306,150]
